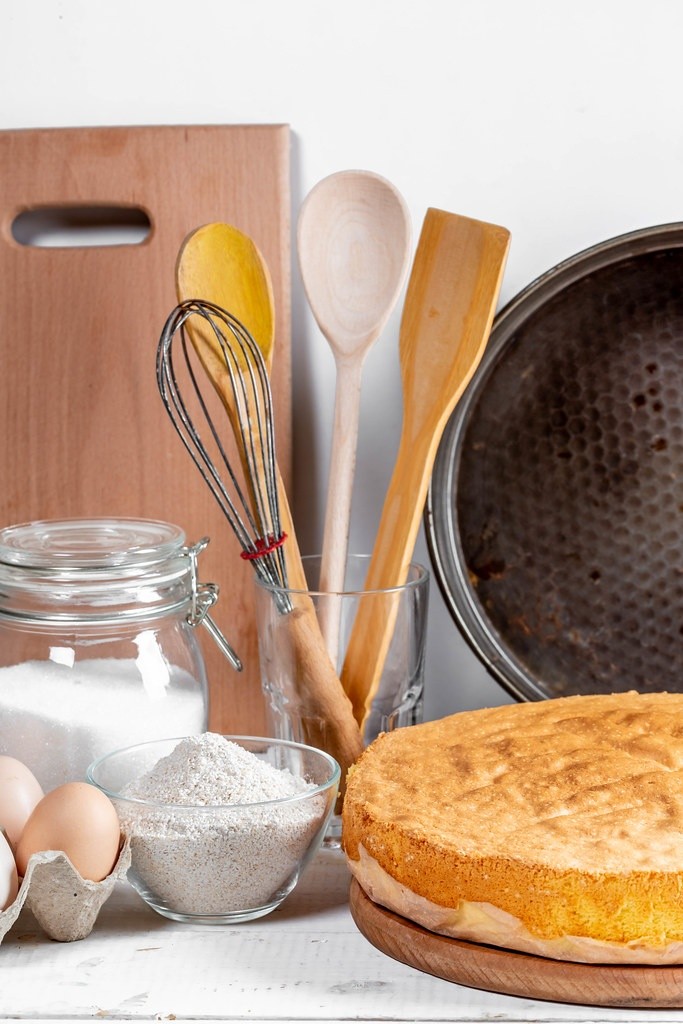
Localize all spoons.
[299,171,410,668]
[176,224,324,750]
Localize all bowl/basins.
[87,736,341,925]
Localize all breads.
[340,690,682,966]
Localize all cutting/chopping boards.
[348,875,683,1007]
[0,122,292,753]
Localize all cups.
[252,553,429,849]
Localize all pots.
[426,222,683,702]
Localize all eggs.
[1,755,121,913]
